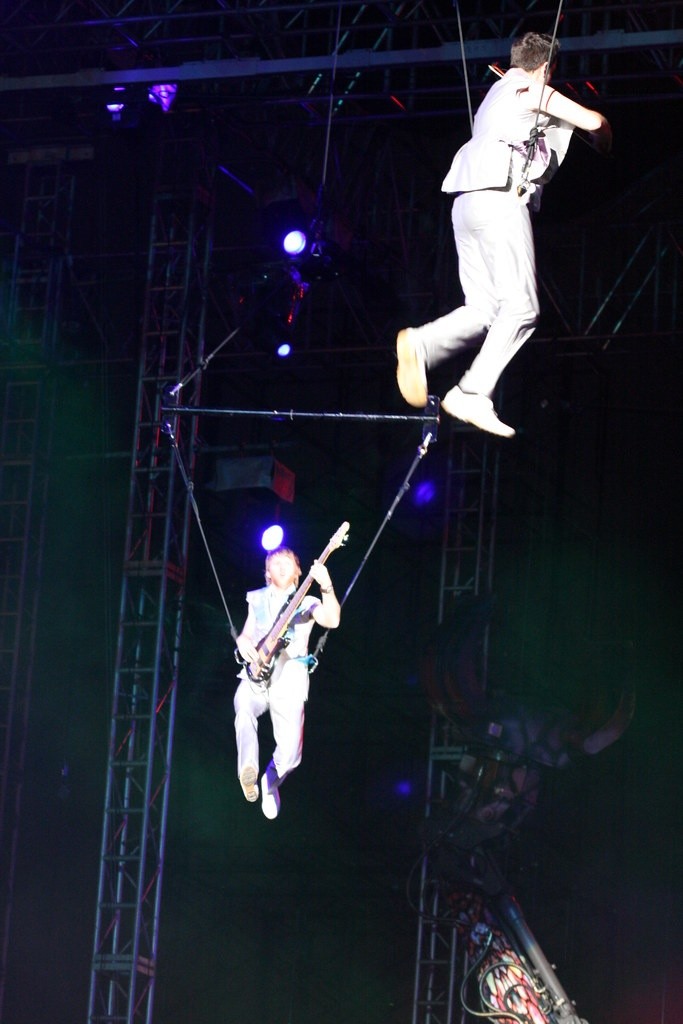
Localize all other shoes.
[239,760,260,802]
[261,774,281,819]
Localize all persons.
[232,547,340,819]
[396,29,610,436]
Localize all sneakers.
[397,327,429,406]
[442,387,515,437]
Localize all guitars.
[234,521,350,698]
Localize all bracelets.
[320,585,333,593]
